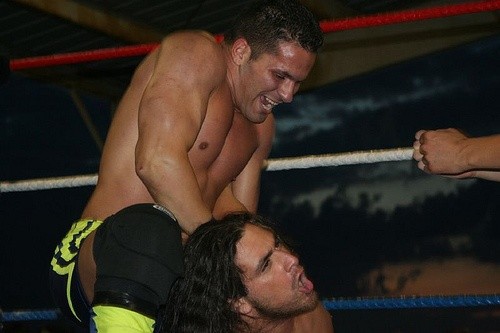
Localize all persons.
[412,128,500,183]
[48,0,323,332]
[153,212,334,333]
